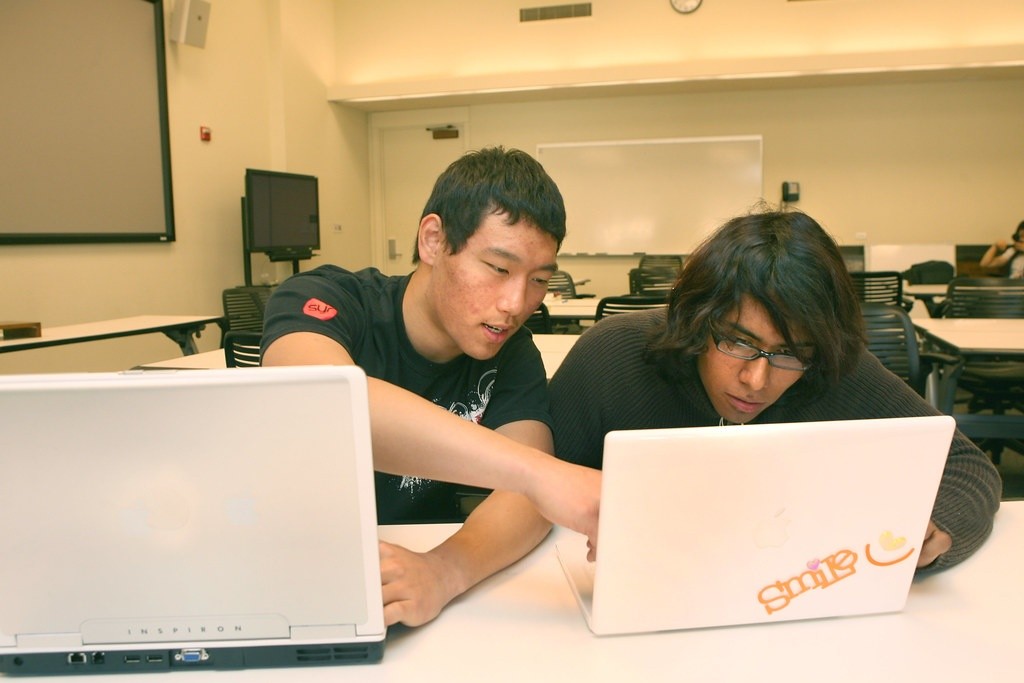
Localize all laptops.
[0,365,389,676]
[552,415,956,636]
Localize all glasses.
[706,315,813,371]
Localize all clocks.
[670,0,703,14]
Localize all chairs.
[222,287,271,368]
[524,254,683,335]
[838,245,1024,501]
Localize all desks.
[883,284,1024,318]
[534,297,669,321]
[0,502,1024,683]
[0,315,223,356]
[910,317,1024,415]
[532,334,581,380]
[139,345,260,369]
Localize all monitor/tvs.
[244,168,321,253]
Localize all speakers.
[167,0,211,48]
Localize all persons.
[978,220,1024,278]
[260,146,604,626]
[547,212,1001,581]
